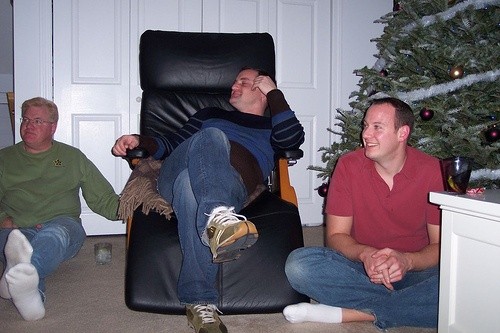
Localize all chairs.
[124,29,312,314]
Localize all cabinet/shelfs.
[430,188,500,333]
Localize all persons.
[111,64,305,333]
[0,97,121,321]
[283,97,444,330]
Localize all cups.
[94,243,112,265]
[442,156,474,195]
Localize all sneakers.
[183,304,228,333]
[206,206,258,264]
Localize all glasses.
[20,118,55,126]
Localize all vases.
[447,156,481,194]
